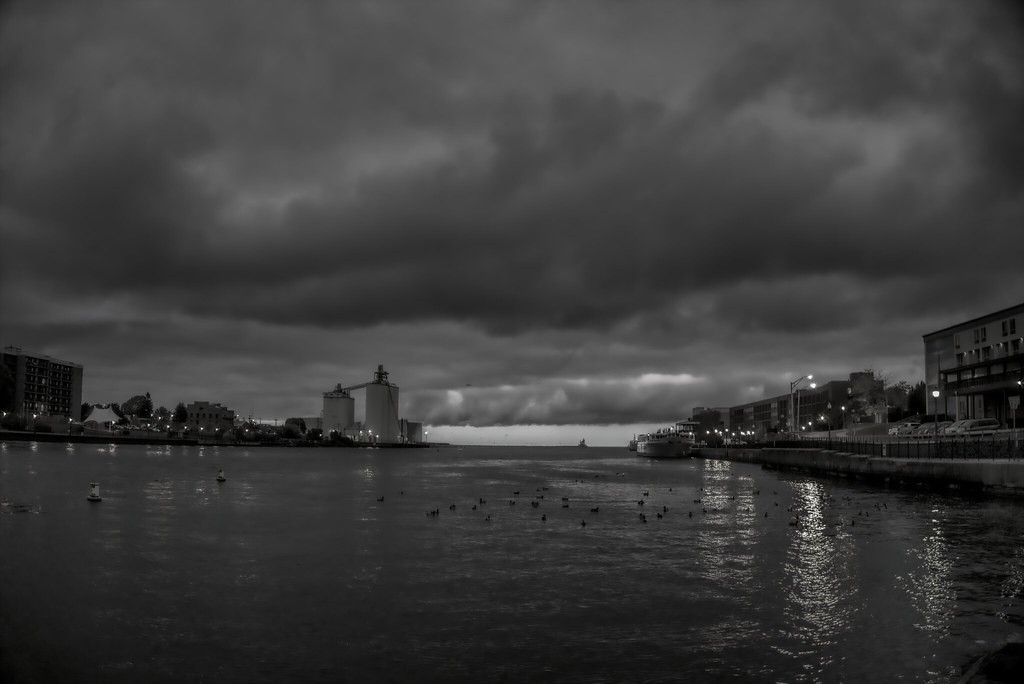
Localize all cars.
[888,418,1001,437]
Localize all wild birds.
[377,495,385,502]
[471,498,491,521]
[401,491,404,495]
[427,508,440,518]
[449,504,455,510]
[509,472,625,528]
[638,472,889,538]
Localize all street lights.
[725,428,728,450]
[931,388,942,452]
[796,383,818,432]
[33,414,37,432]
[199,426,207,443]
[703,429,710,448]
[69,417,73,436]
[751,431,756,448]
[787,374,813,436]
[147,421,155,439]
[739,428,746,445]
[713,428,720,450]
[112,420,116,437]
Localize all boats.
[216,477,227,483]
[636,425,696,459]
[87,482,103,502]
